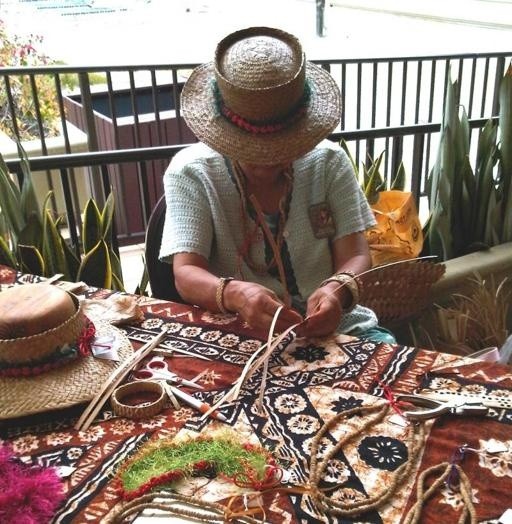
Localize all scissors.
[131,360,205,389]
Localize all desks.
[0,264,512,524]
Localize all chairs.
[145,193,180,303]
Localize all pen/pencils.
[161,382,231,424]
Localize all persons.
[157,27,396,344]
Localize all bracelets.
[319,270,364,313]
[216,276,239,317]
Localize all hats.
[0,283,133,419]
[179,27,342,164]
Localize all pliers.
[396,394,488,419]
